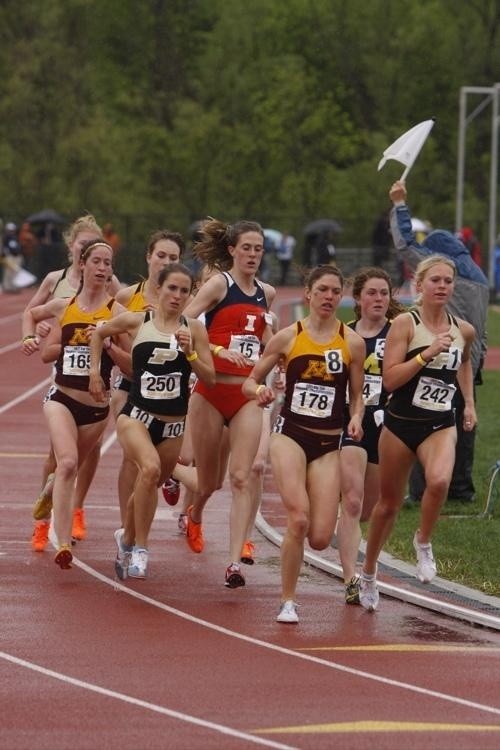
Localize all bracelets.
[417,353,427,366]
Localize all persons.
[458,226,500,293]
[259,219,338,284]
[243,265,366,623]
[371,210,392,268]
[390,181,490,503]
[359,256,477,611]
[3,210,118,289]
[339,268,406,604]
[22,215,286,588]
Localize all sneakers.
[224,562,246,589]
[276,600,301,624]
[358,561,380,613]
[186,504,205,554]
[412,528,437,584]
[54,543,74,570]
[71,507,88,541]
[241,541,256,565]
[161,455,185,506]
[177,514,188,536]
[127,544,149,580]
[31,518,51,552]
[113,527,133,581]
[343,575,360,604]
[403,494,422,507]
[33,472,56,521]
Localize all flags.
[376,117,436,172]
